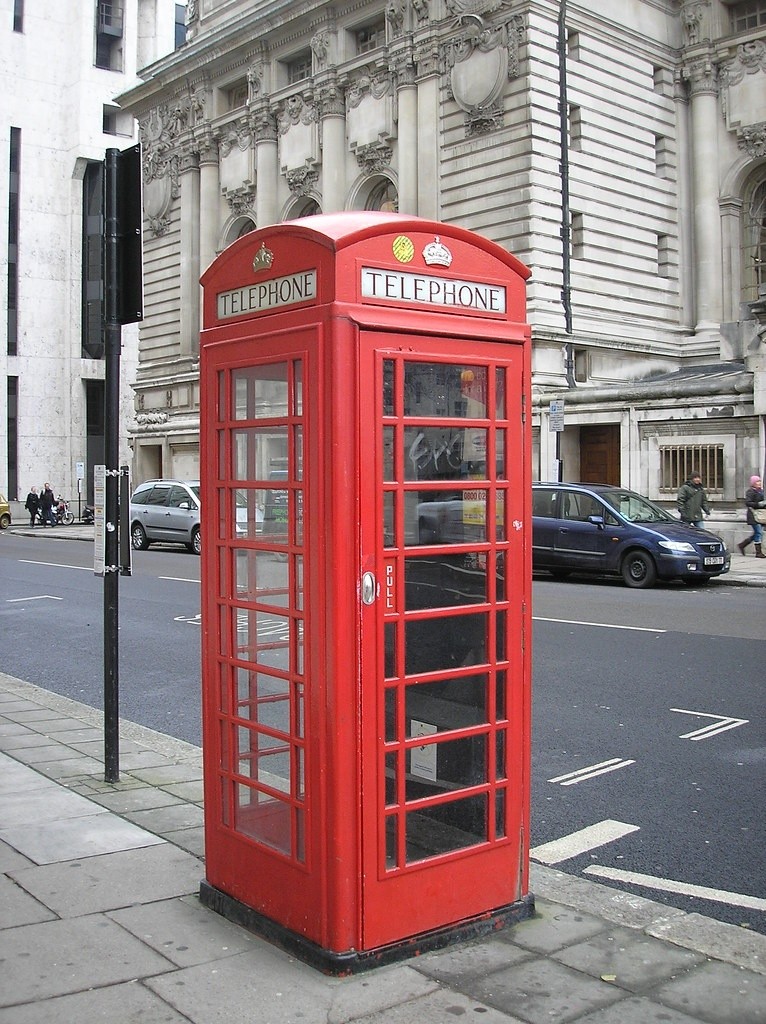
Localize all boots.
[737,538,752,556]
[754,541,766,558]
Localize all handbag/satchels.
[749,507,766,524]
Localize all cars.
[415,492,508,570]
[531,479,730,588]
[128,479,266,556]
[0,495,11,530]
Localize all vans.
[262,471,304,561]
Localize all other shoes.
[43,524,47,528]
[51,522,57,528]
[30,524,35,529]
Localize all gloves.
[705,510,710,515]
[680,511,689,520]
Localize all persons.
[23,486,41,527]
[737,475,766,558]
[40,482,59,527]
[677,470,710,529]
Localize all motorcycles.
[36,494,75,526]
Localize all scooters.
[82,505,94,522]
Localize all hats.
[750,475,762,487]
[688,471,700,480]
